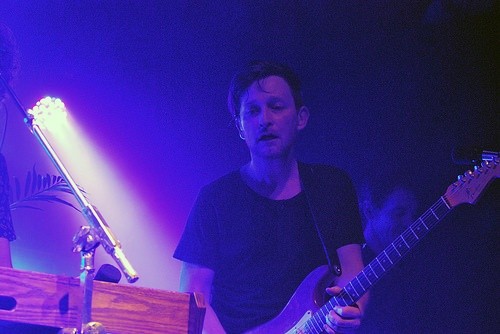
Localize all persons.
[0,24,16,269]
[173,59,368,334]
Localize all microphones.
[93,264,122,283]
[451,140,500,164]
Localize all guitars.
[247,151,500,334]
